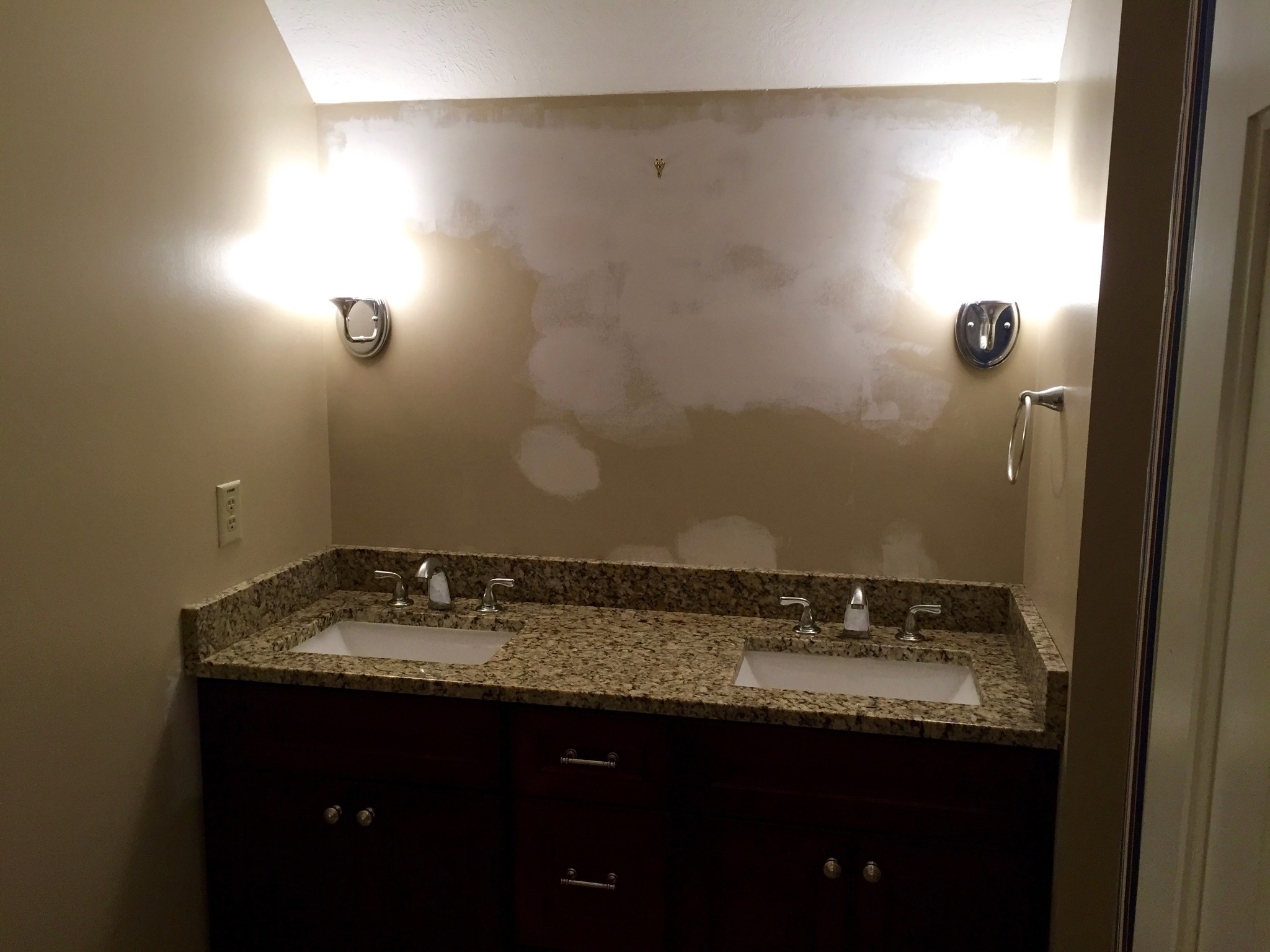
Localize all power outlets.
[214,478,245,550]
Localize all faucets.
[837,582,872,641]
[416,557,456,611]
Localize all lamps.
[326,287,396,367]
[954,294,1024,371]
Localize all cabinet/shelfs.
[193,677,1064,952]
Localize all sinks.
[271,603,527,667]
[730,631,987,709]
